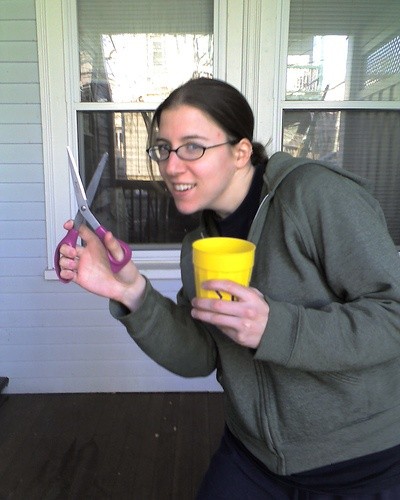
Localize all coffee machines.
[54,146,131,282]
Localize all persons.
[59,78,400,499]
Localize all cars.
[192,237,256,302]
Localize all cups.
[146,140,236,162]
